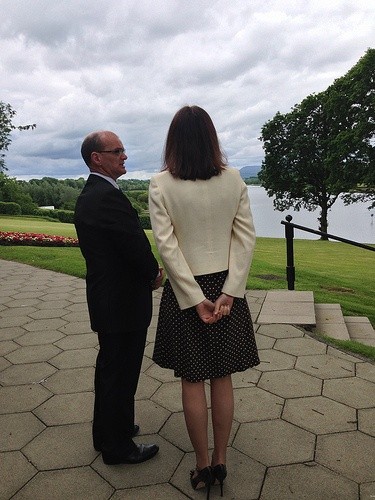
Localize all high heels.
[212,464,228,497]
[190,465,215,500]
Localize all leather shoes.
[95,424,139,451]
[103,444,159,465]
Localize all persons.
[148,105,257,500]
[72,131,165,466]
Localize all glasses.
[97,148,125,155]
